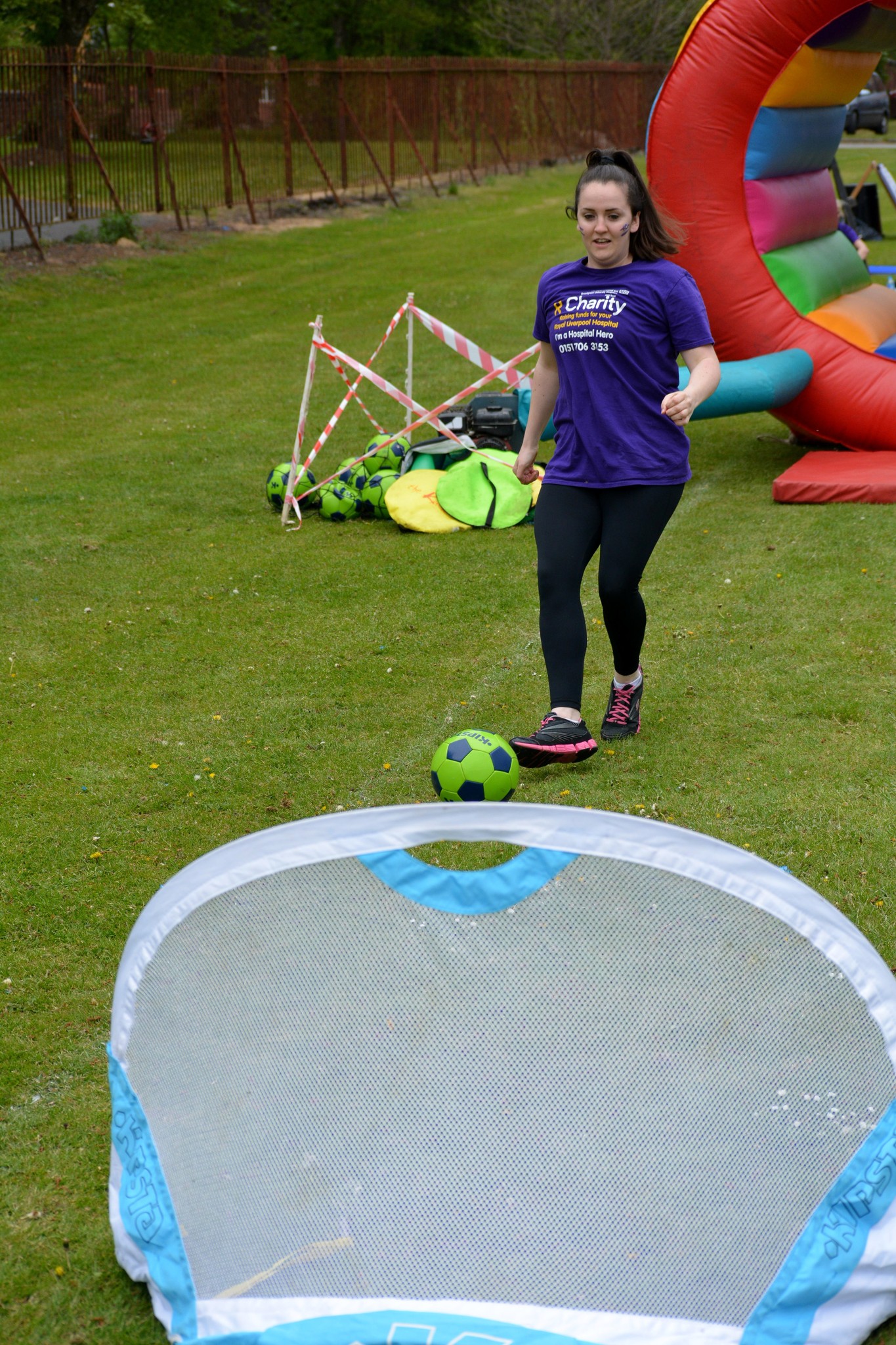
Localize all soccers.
[316,433,412,522]
[429,728,519,807]
[266,462,317,511]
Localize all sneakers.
[509,712,598,768]
[600,664,643,741]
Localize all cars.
[841,72,890,136]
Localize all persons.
[835,200,869,261]
[508,148,720,768]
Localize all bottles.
[886,276,895,290]
